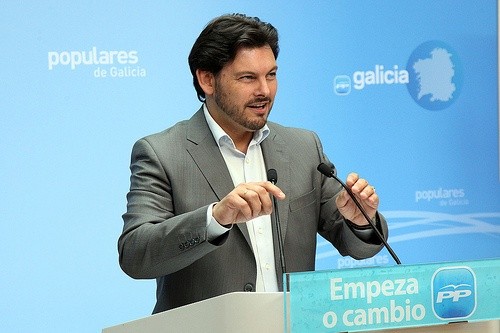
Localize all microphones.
[266,168,286,272]
[317,163,401,265]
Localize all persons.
[118,13,388,315]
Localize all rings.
[368,185,375,192]
[243,189,249,197]
